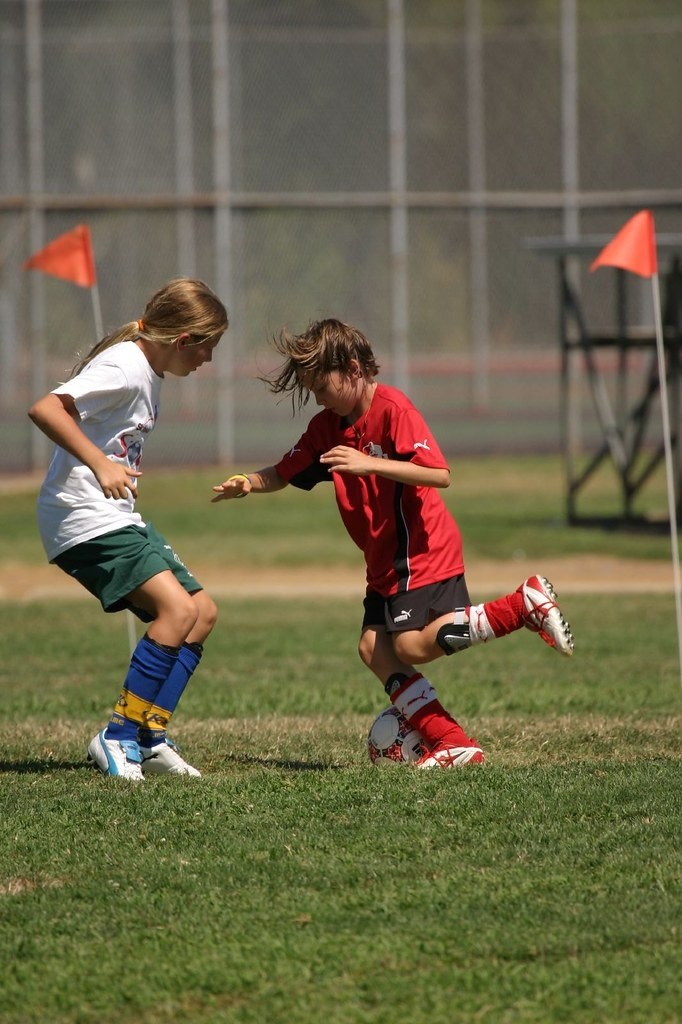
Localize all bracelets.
[228,473,251,484]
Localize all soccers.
[369,707,428,763]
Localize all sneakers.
[88,727,145,781]
[413,737,486,770]
[515,575,574,658]
[140,738,202,779]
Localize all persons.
[212,319,574,771]
[27,279,228,781]
